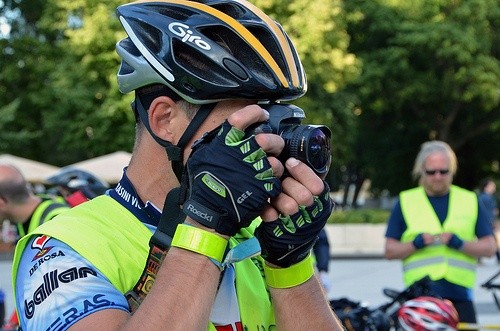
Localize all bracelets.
[170,224,228,262]
[264,250,315,288]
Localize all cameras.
[244,102,333,181]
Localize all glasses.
[425,169,449,176]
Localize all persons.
[0,162,73,236]
[478,180,500,264]
[12,1,347,330]
[383,143,498,325]
[313,228,331,295]
[43,168,107,207]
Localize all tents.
[0,154,60,189]
[70,150,132,182]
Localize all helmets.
[116,0,308,105]
[397,296,459,331]
[41,169,107,200]
